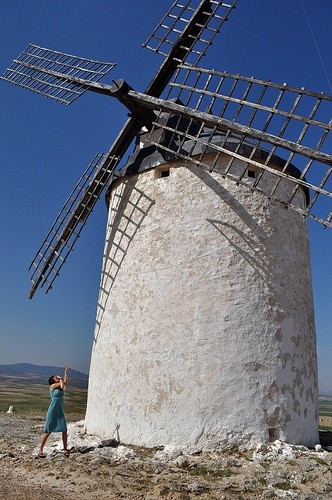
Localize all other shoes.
[38,452,46,458]
[63,450,70,457]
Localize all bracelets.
[59,378,62,380]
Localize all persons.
[38,363,71,457]
